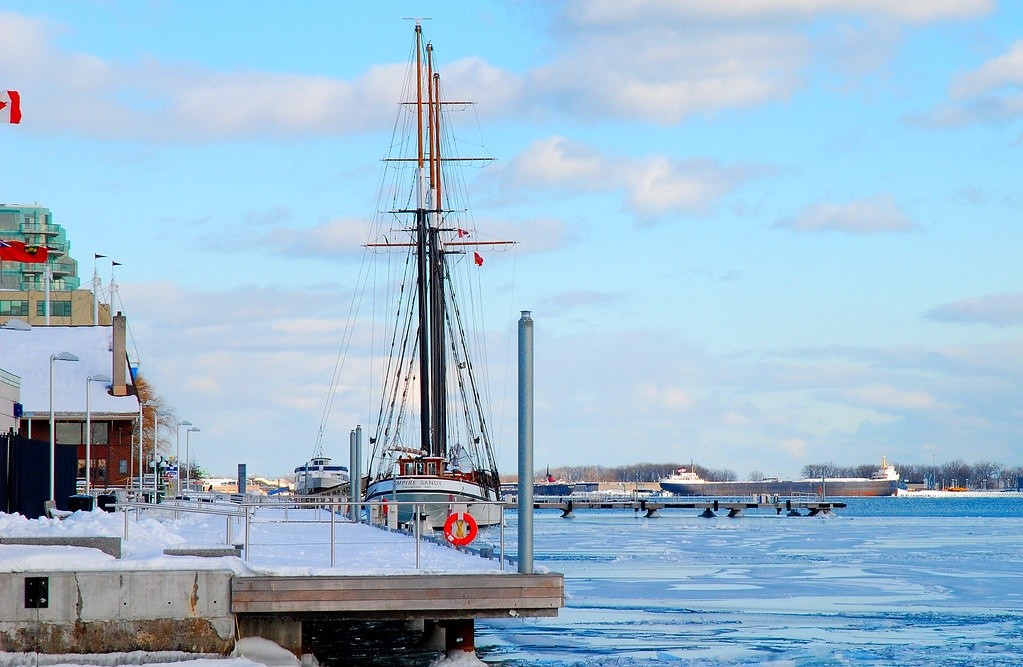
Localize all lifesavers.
[381,498,387,514]
[444,513,478,547]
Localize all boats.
[496,478,577,496]
[659,455,899,498]
[290,433,351,514]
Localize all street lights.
[153,410,171,503]
[49,352,80,501]
[176,421,192,497]
[185,426,201,498]
[635,473,639,499]
[139,400,158,501]
[86,373,110,497]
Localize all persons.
[208,485,213,491]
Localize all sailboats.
[303,17,508,531]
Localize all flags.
[0,239,48,263]
[473,252,484,267]
[0,89,22,124]
[457,229,469,238]
